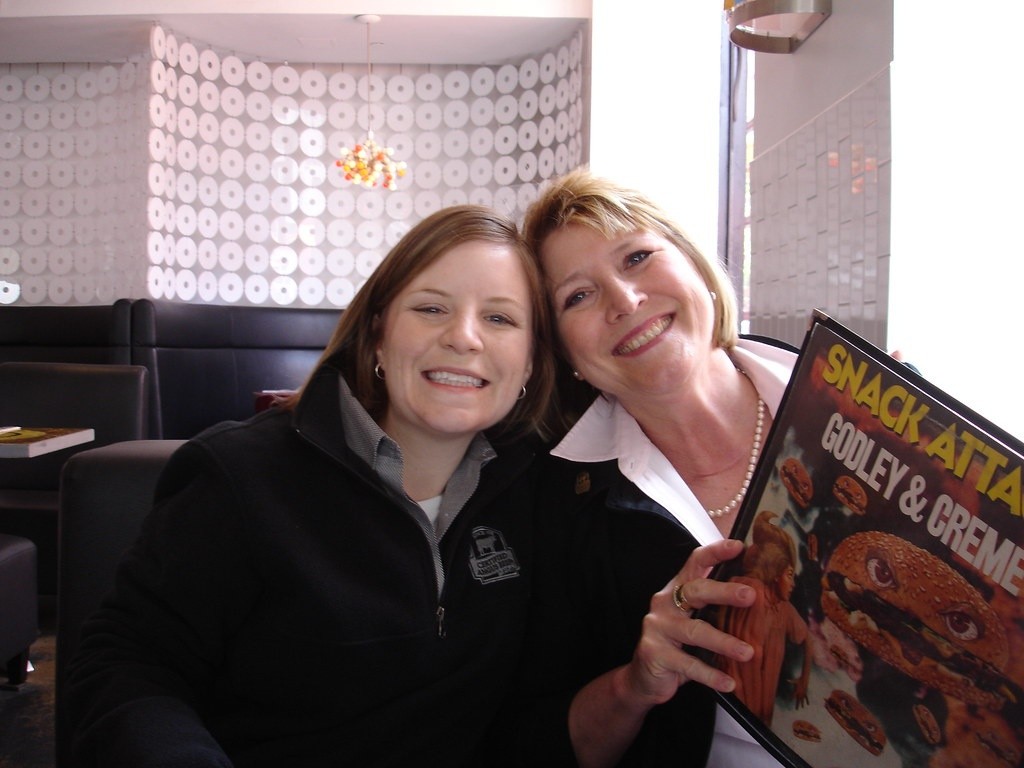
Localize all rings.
[673,583,690,611]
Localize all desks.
[0,425,96,458]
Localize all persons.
[490,168,921,768]
[70,206,558,768]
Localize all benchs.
[0,297,346,768]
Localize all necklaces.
[706,367,765,519]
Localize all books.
[682,306,1024,768]
[0,424,95,460]
